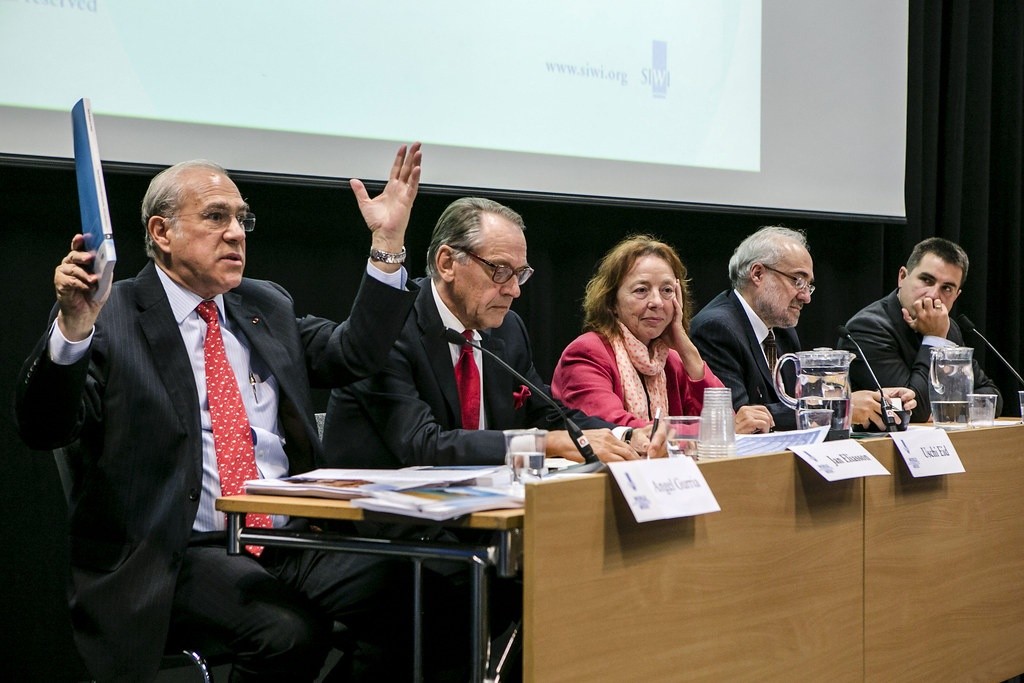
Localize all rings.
[936,306,942,309]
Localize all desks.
[216,418,1024,682]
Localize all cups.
[1018,391,1024,422]
[966,393,998,427]
[663,416,701,460]
[503,429,549,489]
[697,387,736,461]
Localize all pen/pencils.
[647,407,661,459]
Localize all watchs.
[370,246,407,264]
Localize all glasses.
[457,248,534,285]
[765,265,816,295]
[164,209,256,233]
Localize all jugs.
[772,346,857,437]
[928,345,974,427]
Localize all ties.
[455,329,481,430]
[195,300,274,558]
[763,332,779,387]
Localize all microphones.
[835,325,897,433]
[438,326,604,474]
[956,313,1024,386]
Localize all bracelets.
[624,429,633,445]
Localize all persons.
[550,236,775,454]
[687,227,916,432]
[11,143,422,683]
[321,196,679,470]
[837,237,1003,423]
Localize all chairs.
[53,441,357,683]
